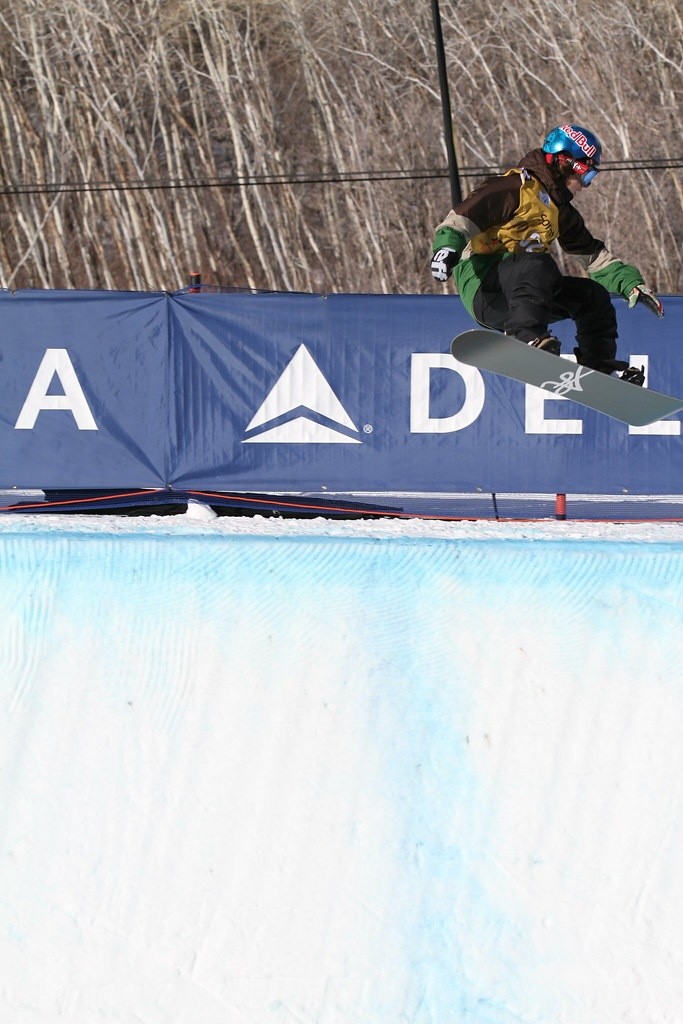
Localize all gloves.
[627,285,665,318]
[431,247,460,281]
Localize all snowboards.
[450,328,683,427]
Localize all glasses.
[567,158,599,188]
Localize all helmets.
[541,123,601,167]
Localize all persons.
[431,124,665,385]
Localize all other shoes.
[607,366,644,386]
[527,334,561,355]
